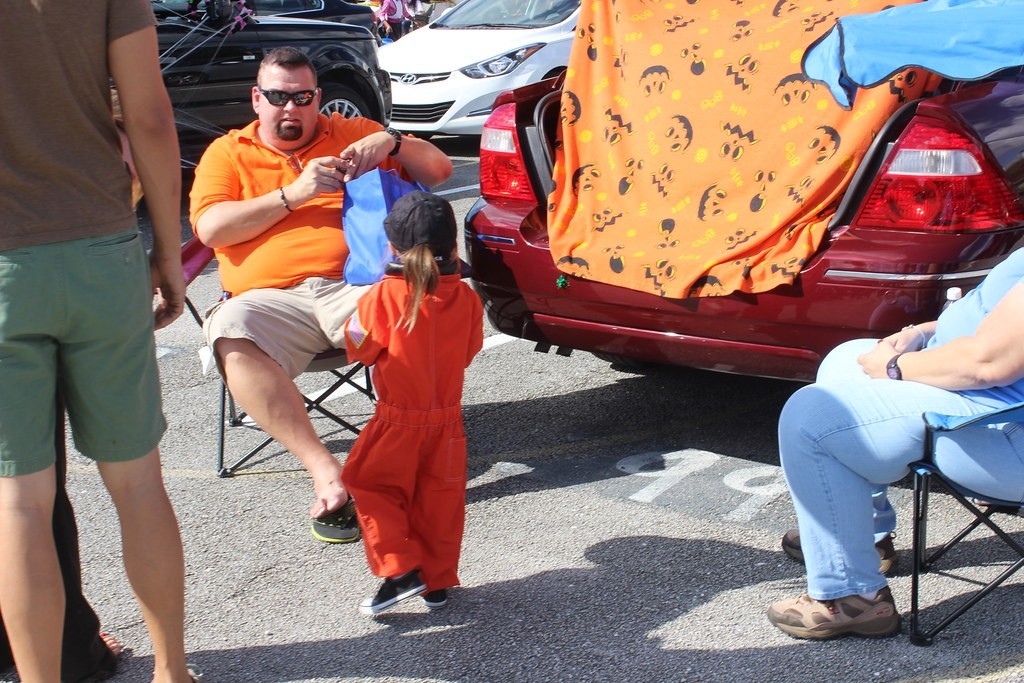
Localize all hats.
[383,191,457,252]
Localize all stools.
[215,298,377,475]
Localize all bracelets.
[903,325,924,348]
[385,127,401,156]
[280,184,294,212]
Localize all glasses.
[260,89,317,106]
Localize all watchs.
[887,355,902,379]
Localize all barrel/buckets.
[381,38,393,45]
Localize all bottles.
[941,287,962,313]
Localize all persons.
[0,0,198,683]
[377,0,455,49]
[341,191,483,618]
[190,46,454,521]
[767,245,1023,639]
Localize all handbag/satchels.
[342,166,432,285]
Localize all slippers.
[313,500,359,543]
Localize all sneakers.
[359,569,427,615]
[768,585,901,641]
[784,529,899,576]
[422,588,449,607]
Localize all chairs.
[906,403,1024,649]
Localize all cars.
[110,0,393,172]
[378,0,581,140]
[465,65,1024,384]
